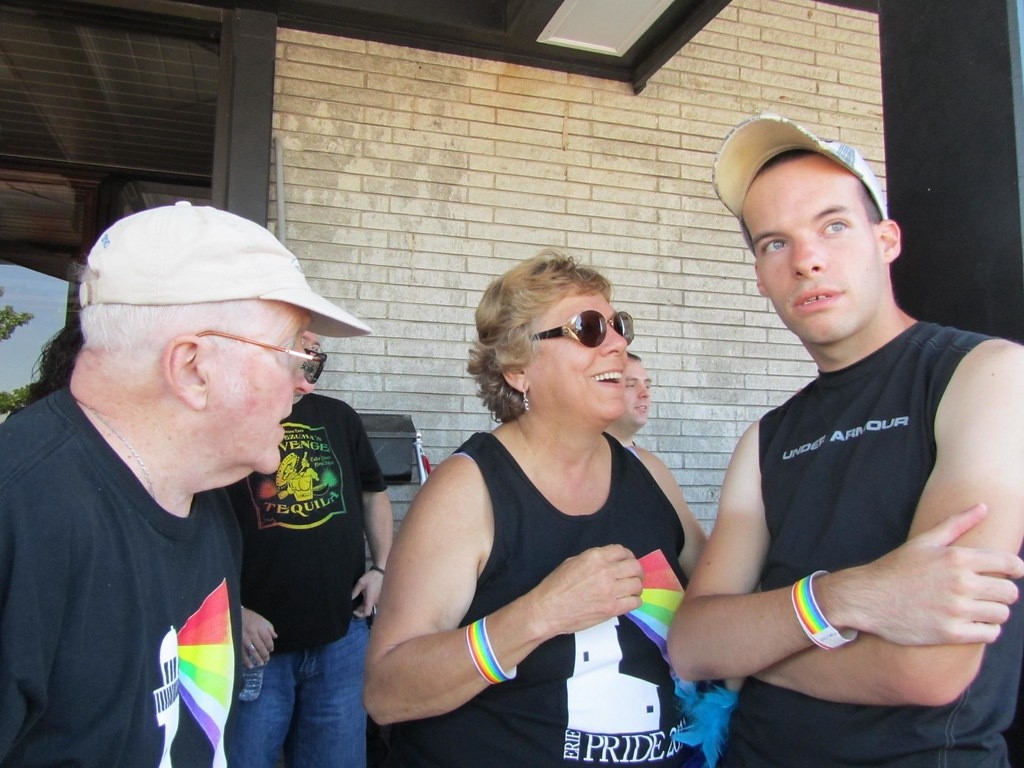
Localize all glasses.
[179,328,327,386]
[527,310,638,349]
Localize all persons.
[664,116,1024,768]
[0,197,396,768]
[605,352,653,447]
[360,251,744,768]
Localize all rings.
[247,644,255,651]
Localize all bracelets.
[370,566,384,574]
[791,571,857,650]
[465,618,516,684]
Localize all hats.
[79,196,372,339]
[711,113,891,260]
